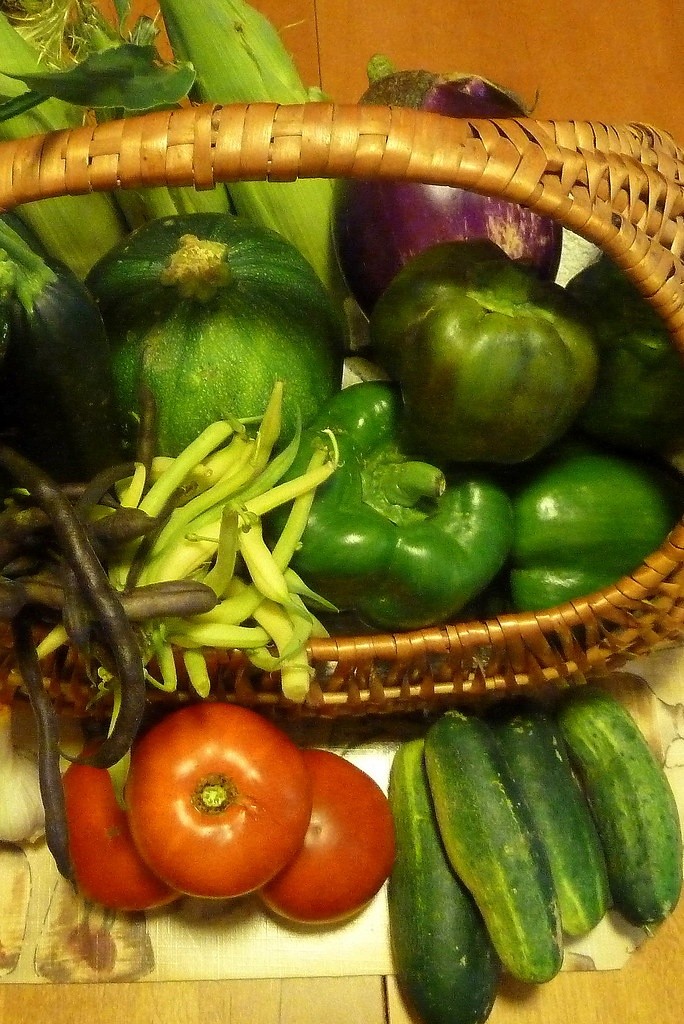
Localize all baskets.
[2,101,684,718]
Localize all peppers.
[268,236,682,632]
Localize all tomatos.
[59,704,395,924]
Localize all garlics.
[0,701,52,844]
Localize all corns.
[1,0,339,290]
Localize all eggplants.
[332,52,561,313]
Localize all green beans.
[0,381,342,736]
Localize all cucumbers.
[386,686,683,1023]
